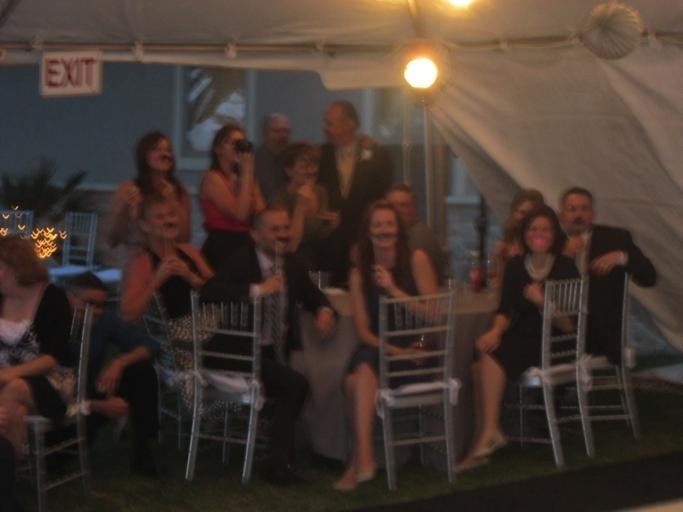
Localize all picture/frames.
[174,65,255,171]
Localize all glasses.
[69,288,108,310]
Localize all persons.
[194,123,265,311]
[107,131,191,280]
[332,197,439,493]
[449,205,582,473]
[265,141,339,245]
[118,195,216,336]
[198,205,337,482]
[385,183,444,286]
[511,186,657,453]
[486,187,544,292]
[262,112,292,182]
[0,233,80,512]
[310,100,393,288]
[44,271,164,477]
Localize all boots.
[0,436,26,512]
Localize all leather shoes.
[470,429,505,459]
[453,454,490,472]
[264,456,320,485]
[131,455,171,479]
[333,466,357,492]
[355,455,378,483]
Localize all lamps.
[396,41,451,96]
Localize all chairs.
[24,302,95,511]
[336,290,461,492]
[0,210,33,236]
[142,312,194,449]
[546,271,642,441]
[503,276,598,472]
[185,293,278,486]
[61,211,99,267]
